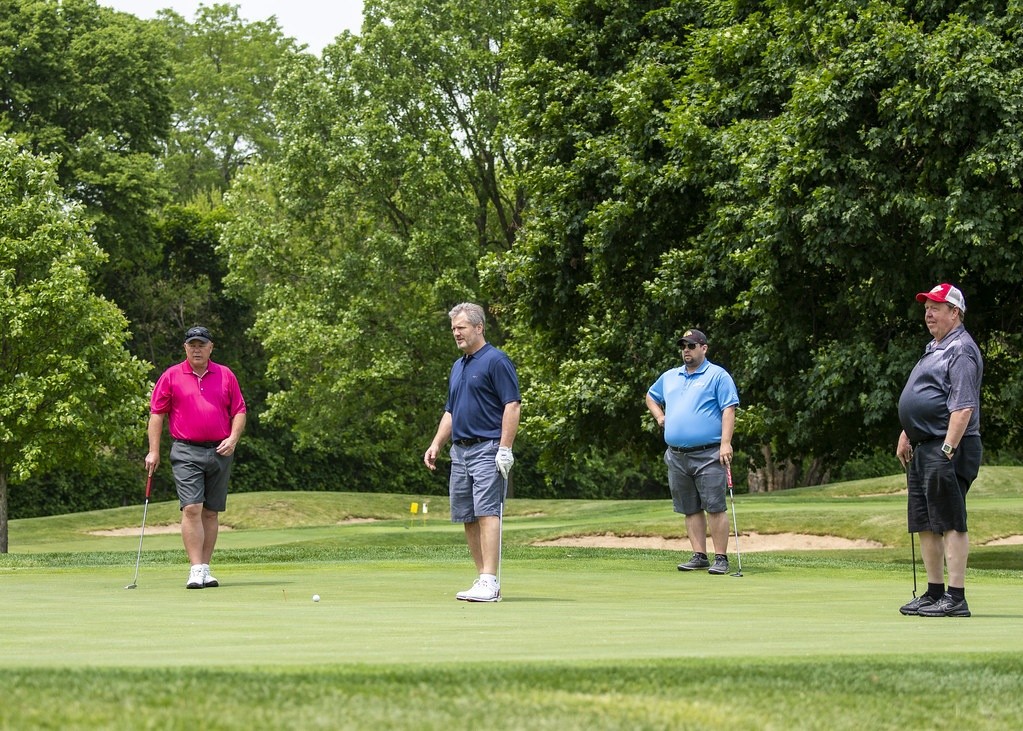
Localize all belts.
[670,445,715,453]
[177,439,220,449]
[454,438,491,446]
[918,437,945,446]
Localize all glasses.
[679,343,695,350]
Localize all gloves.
[495,447,514,480]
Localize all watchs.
[940,442,957,454]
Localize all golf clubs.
[497,472,505,602]
[125,469,154,590]
[903,459,917,600]
[726,457,744,577]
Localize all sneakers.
[186,564,204,589]
[678,553,710,571]
[466,580,500,602]
[899,595,936,616]
[917,593,971,617]
[456,579,479,600]
[708,555,730,575]
[202,564,218,587]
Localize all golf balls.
[313,594,320,602]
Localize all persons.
[645,328,739,575]
[145,326,246,589]
[424,302,522,603]
[897,284,983,618]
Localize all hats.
[184,327,209,344]
[916,283,966,313]
[677,329,706,346]
